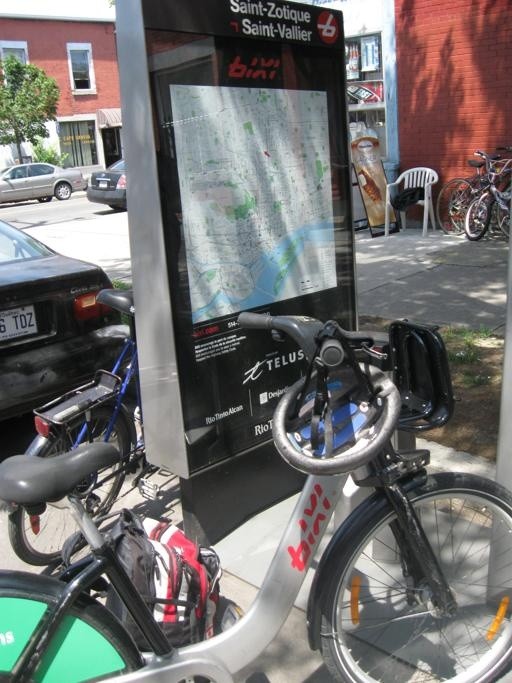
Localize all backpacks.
[101,508,223,653]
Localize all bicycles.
[428,146,512,243]
[0,293,512,682]
[5,284,145,569]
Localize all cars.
[0,161,88,203]
[82,157,126,211]
[0,217,127,442]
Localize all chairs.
[384,166,439,237]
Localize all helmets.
[272,359,403,478]
